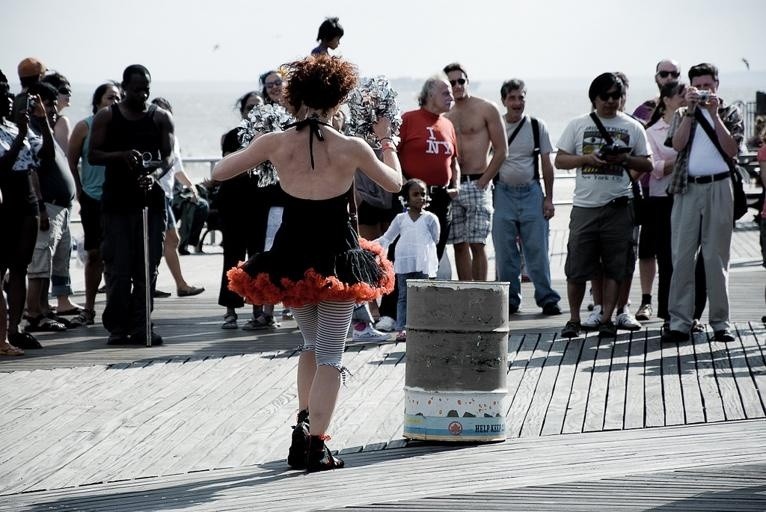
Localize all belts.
[460,175,483,182]
[688,171,730,183]
[44,198,70,207]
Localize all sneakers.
[352,316,396,342]
[543,303,734,342]
[450,79,465,86]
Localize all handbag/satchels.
[733,173,747,220]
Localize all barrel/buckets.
[403,279,511,443]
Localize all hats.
[18,57,49,76]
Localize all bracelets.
[379,136,397,152]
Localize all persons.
[442,65,508,282]
[73,81,209,326]
[755,131,766,327]
[240,17,350,332]
[209,54,403,470]
[1,57,74,356]
[324,105,393,342]
[581,59,716,333]
[89,65,174,346]
[216,90,267,328]
[662,65,744,343]
[376,76,462,332]
[488,80,560,315]
[555,73,654,338]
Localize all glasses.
[58,88,71,94]
[656,70,680,78]
[266,80,282,88]
[600,92,620,100]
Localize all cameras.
[20,93,36,112]
[600,142,633,164]
[135,149,164,174]
[692,89,713,105]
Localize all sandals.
[0,287,293,356]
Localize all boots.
[288,410,310,466]
[307,434,344,471]
